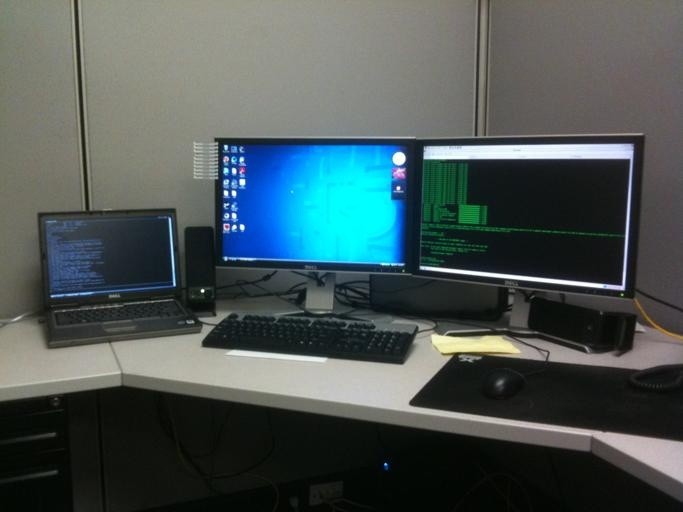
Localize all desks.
[0,293,682,511]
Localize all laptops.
[37,207,203,348]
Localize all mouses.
[479,367,525,399]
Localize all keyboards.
[202,313,418,365]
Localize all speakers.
[369,274,508,322]
[184,225,217,316]
[527,292,639,357]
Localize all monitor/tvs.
[212,136,417,319]
[411,133,645,354]
